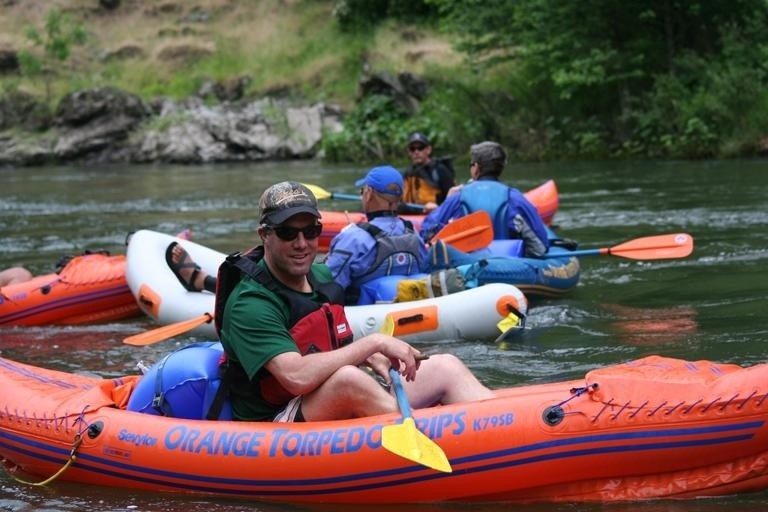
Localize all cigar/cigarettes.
[401,354,429,362]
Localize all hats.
[354,166,403,195]
[259,182,322,224]
[406,133,429,146]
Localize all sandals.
[166,242,203,292]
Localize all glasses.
[409,145,425,152]
[265,222,322,240]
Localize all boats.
[0,230,197,326]
[424,226,584,295]
[124,230,529,347]
[316,175,557,252]
[0,350,768,501]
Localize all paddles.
[423,210,492,253]
[382,319,455,476]
[543,233,692,261]
[297,183,427,209]
[123,312,214,347]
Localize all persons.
[420,141,550,258]
[166,165,429,306]
[219,178,498,423]
[402,133,455,213]
